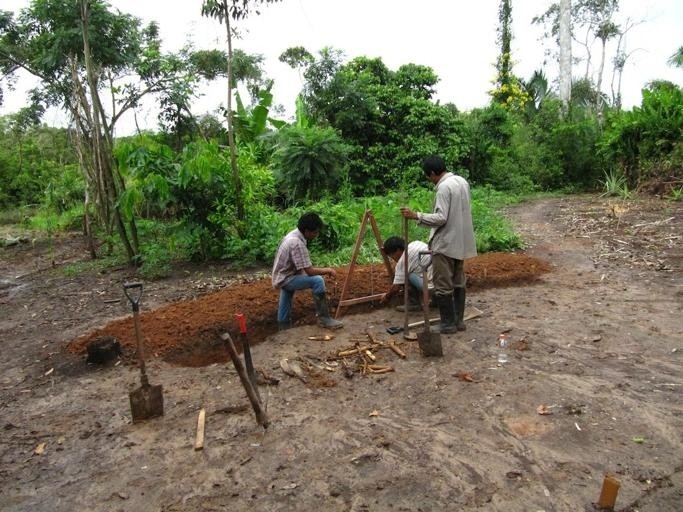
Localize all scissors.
[387,251,482,358]
[122,284,164,424]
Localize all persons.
[397,155,478,335]
[377,236,438,311]
[270,212,344,331]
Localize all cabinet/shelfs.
[498,334,509,363]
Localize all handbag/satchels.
[386,294,391,299]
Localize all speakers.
[428,291,459,333]
[395,281,422,312]
[312,291,344,329]
[453,287,469,330]
